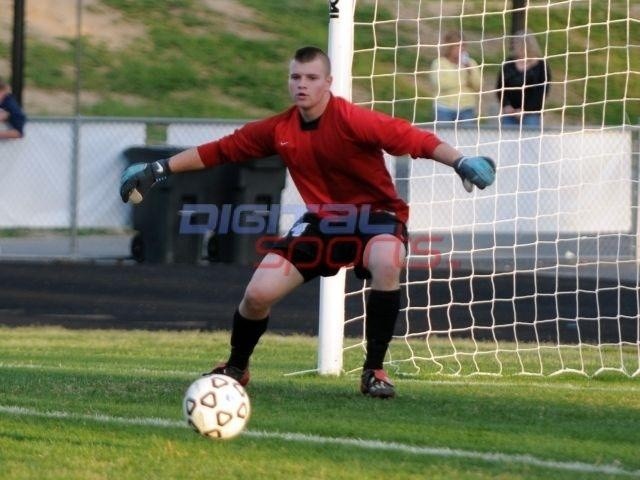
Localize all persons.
[121,47,496,397]
[429,31,483,124]
[497,28,552,125]
[1,78,24,139]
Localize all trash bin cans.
[122,146,204,266]
[195,152,287,264]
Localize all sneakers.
[360,367,395,397]
[203,364,250,388]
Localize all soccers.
[183,375,251,439]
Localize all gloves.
[118,158,168,204]
[453,154,497,194]
